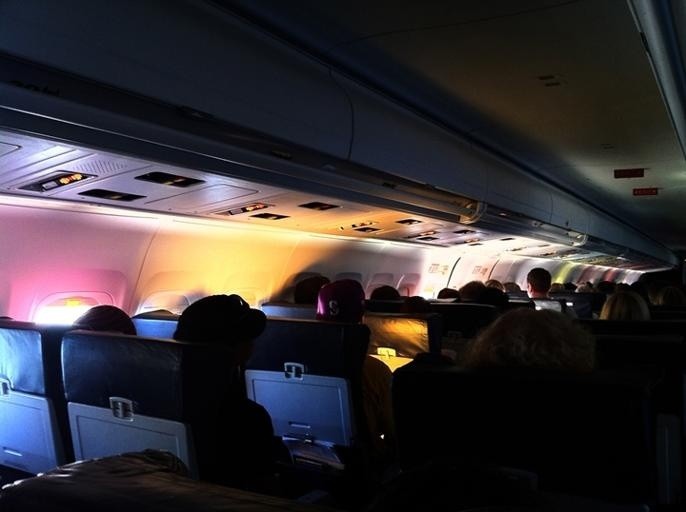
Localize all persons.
[70,265,649,499]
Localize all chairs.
[0,256,686,511]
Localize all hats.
[173,293,268,344]
[315,278,369,322]
